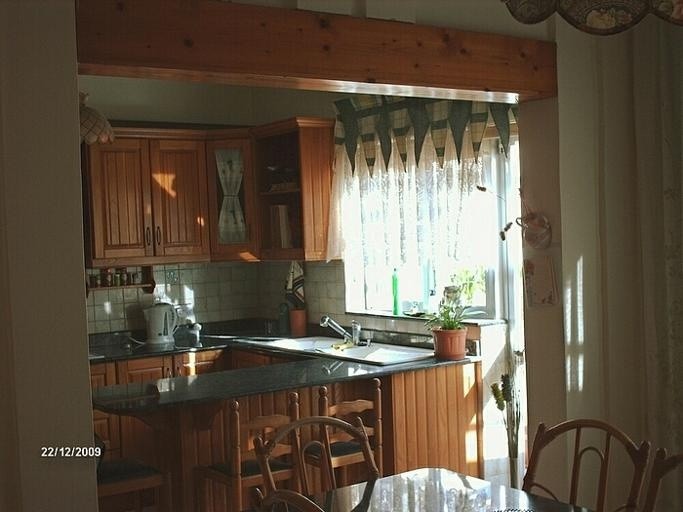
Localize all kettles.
[141,302,178,352]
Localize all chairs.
[522,416,652,511]
[201,391,312,511]
[299,378,384,494]
[244,486,325,512]
[642,444,683,512]
[254,415,381,496]
[94,436,171,511]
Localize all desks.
[241,466,600,512]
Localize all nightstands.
[205,139,258,262]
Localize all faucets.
[320,315,362,344]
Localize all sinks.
[267,337,435,365]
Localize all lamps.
[77,91,115,147]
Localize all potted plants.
[420,300,473,361]
[444,272,475,301]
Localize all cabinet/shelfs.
[86,135,210,270]
[250,129,335,262]
[233,350,484,482]
[115,350,231,512]
[88,359,125,464]
